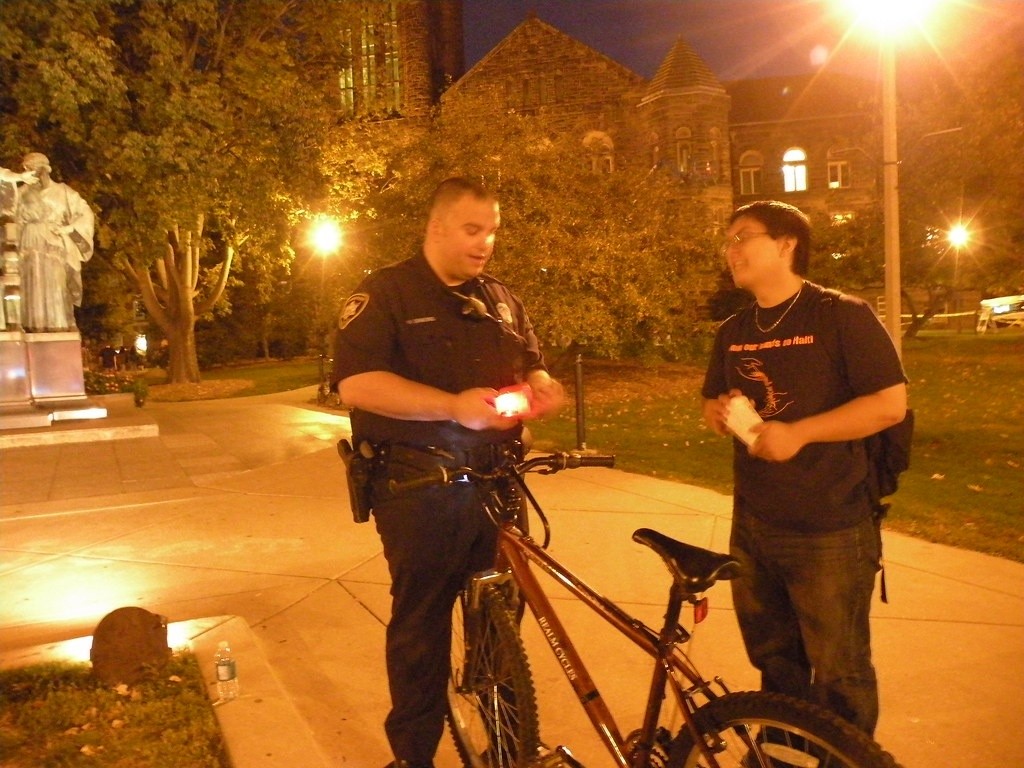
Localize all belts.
[372,438,524,474]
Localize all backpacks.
[819,288,913,498]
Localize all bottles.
[214,642,239,698]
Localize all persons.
[700,201,908,768]
[329,177,567,768]
[0,153,95,332]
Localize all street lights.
[945,224,969,334]
[840,0,941,364]
[307,215,345,388]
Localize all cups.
[722,395,765,448]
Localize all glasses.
[717,231,789,256]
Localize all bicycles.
[359,438,902,768]
[315,354,342,406]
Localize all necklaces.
[756,279,803,332]
